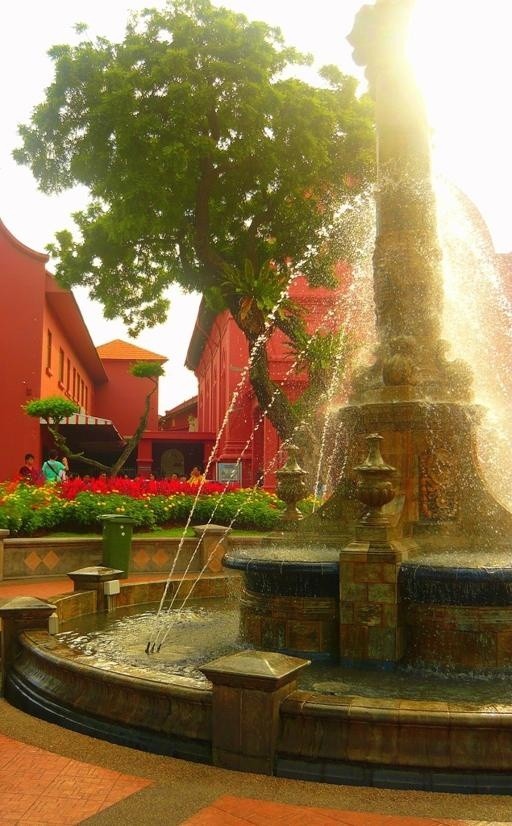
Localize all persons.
[41,449,69,484]
[68,465,205,486]
[19,453,37,485]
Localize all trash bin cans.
[96,514,139,579]
